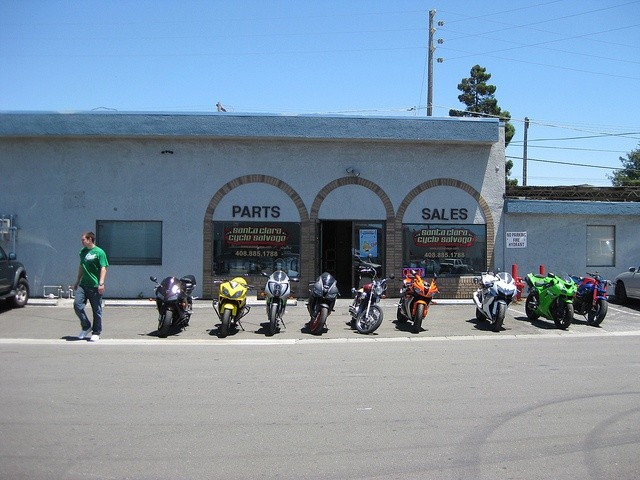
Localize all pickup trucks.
[440,259,468,271]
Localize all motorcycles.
[396,269,440,332]
[525,272,578,328]
[212,276,255,336]
[150,275,197,337]
[472,268,521,331]
[568,272,613,326]
[260,270,299,334]
[306,272,341,335]
[349,253,395,335]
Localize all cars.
[613,264,640,303]
[0,245,30,308]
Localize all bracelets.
[99,284,104,286]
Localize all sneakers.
[90,335,99,342]
[78,327,92,339]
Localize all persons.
[73,233,109,342]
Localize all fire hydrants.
[516,275,525,300]
